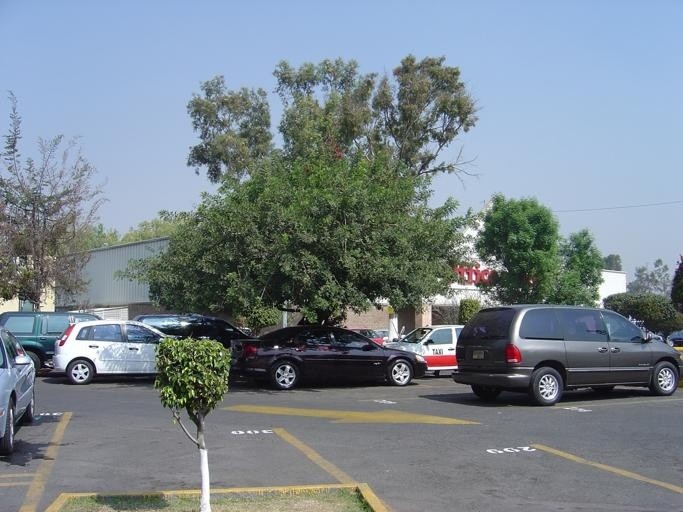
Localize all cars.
[0,326,35,454]
